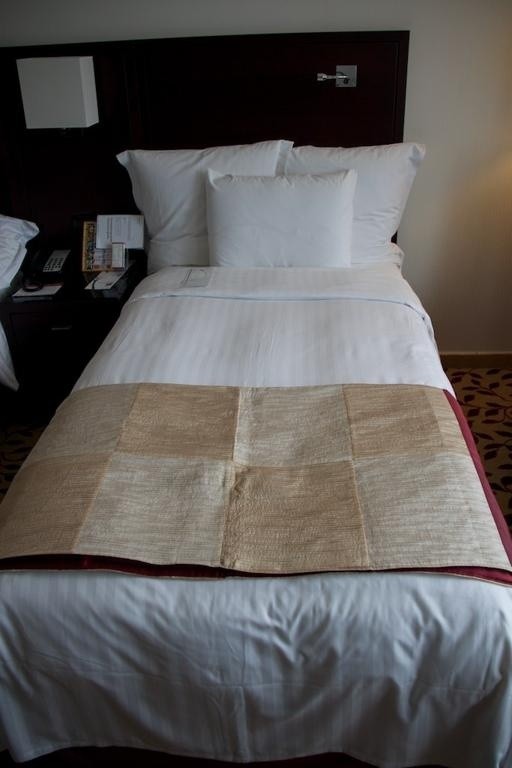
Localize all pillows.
[286,143,428,267]
[0,215,81,289]
[115,139,295,269]
[205,168,358,266]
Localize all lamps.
[15,56,100,136]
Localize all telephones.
[25,244,70,281]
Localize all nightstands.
[0,258,146,421]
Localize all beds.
[0,241,512,768]
